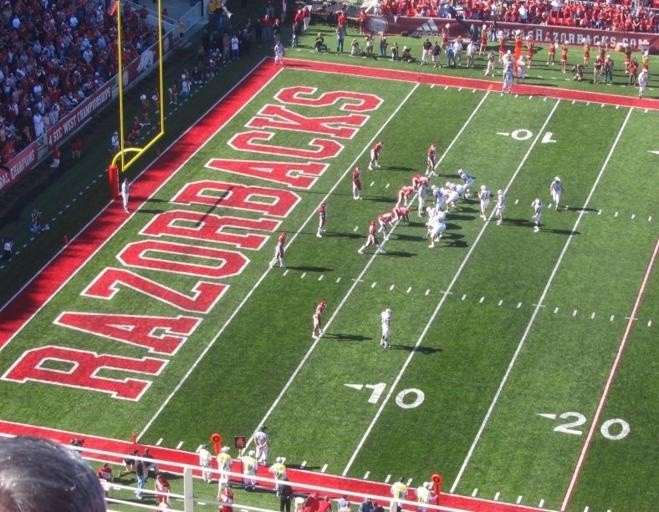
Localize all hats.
[423,482,430,487]
[249,450,255,456]
[222,447,230,452]
[277,458,282,462]
[261,426,266,430]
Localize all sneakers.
[384,237,389,240]
[533,226,539,233]
[445,210,449,213]
[368,164,381,171]
[316,229,325,238]
[434,234,442,242]
[136,495,142,499]
[380,251,386,255]
[312,331,324,339]
[428,244,435,248]
[268,262,274,269]
[497,220,503,226]
[280,265,286,268]
[358,252,365,254]
[389,222,394,228]
[353,196,364,200]
[556,207,559,211]
[419,215,423,218]
[480,215,486,221]
[382,344,393,349]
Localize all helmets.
[445,183,449,186]
[372,222,376,226]
[555,177,560,182]
[282,232,287,238]
[386,309,392,314]
[356,167,360,171]
[426,208,430,213]
[458,169,462,175]
[535,200,540,204]
[321,301,326,306]
[321,203,326,208]
[431,186,435,191]
[481,186,485,191]
[498,190,503,194]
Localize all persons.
[1,0,170,160]
[268,456,287,494]
[122,178,130,213]
[531,197,543,232]
[269,229,286,269]
[357,169,475,255]
[199,444,212,483]
[280,478,293,511]
[253,425,268,466]
[549,176,563,210]
[368,141,383,171]
[317,204,327,238]
[296,476,441,512]
[62,232,68,245]
[426,144,440,177]
[494,189,505,225]
[216,445,233,486]
[169,0,254,108]
[73,438,84,452]
[477,185,490,220]
[117,447,138,479]
[219,483,233,511]
[154,473,171,512]
[352,1,659,99]
[380,308,391,348]
[133,457,145,499]
[260,0,349,65]
[311,299,329,338]
[0,436,105,512]
[97,462,112,497]
[352,168,363,201]
[4,239,12,254]
[30,207,56,235]
[238,448,258,491]
[142,447,153,480]
